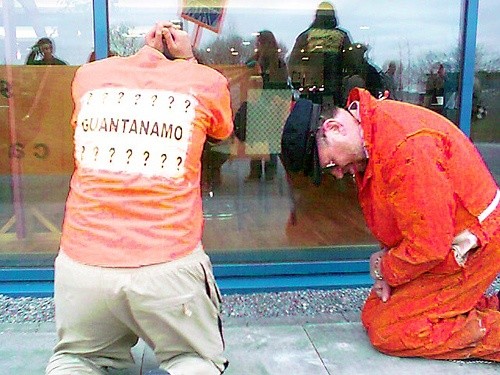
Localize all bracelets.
[373,255,382,280]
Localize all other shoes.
[243,176,260,182]
[265,178,275,183]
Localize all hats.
[281,99,322,188]
[315,2,334,16]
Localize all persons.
[280,87,500,362]
[26,1,482,191]
[42,20,234,375]
